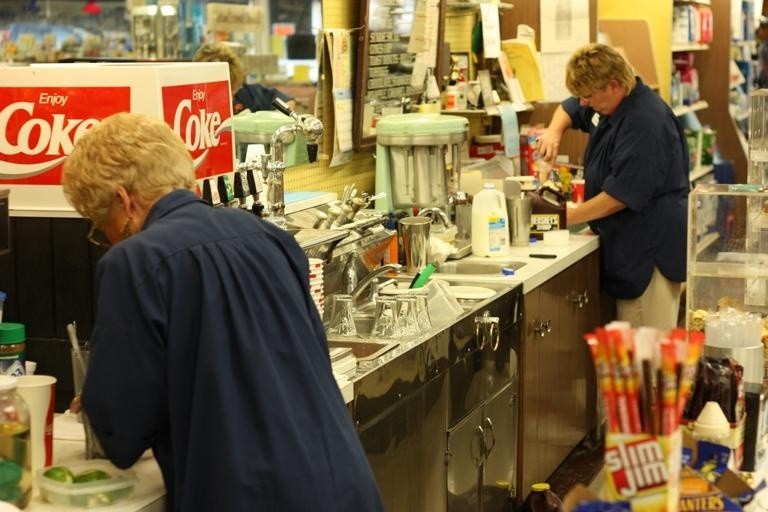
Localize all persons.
[196,40,309,116]
[536,41,690,332]
[59,110,383,512]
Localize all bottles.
[446,73,468,111]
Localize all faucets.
[370,277,400,304]
[415,205,452,229]
[342,249,403,315]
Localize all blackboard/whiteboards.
[362,1,417,147]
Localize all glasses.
[88,219,112,247]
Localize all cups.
[571,179,585,203]
[398,216,432,276]
[0,323,35,509]
[506,195,533,246]
[7,371,58,470]
[330,292,435,341]
[309,259,324,320]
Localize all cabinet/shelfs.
[445,289,521,512]
[517,251,618,506]
[595,0,723,258]
[352,330,448,512]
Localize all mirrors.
[353,0,448,152]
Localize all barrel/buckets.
[471,183,511,256]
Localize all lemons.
[41,465,131,505]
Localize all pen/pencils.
[583,320,704,431]
[408,265,435,288]
[530,254,557,258]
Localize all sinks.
[358,303,474,323]
[327,338,392,360]
[431,255,529,275]
[335,316,382,336]
